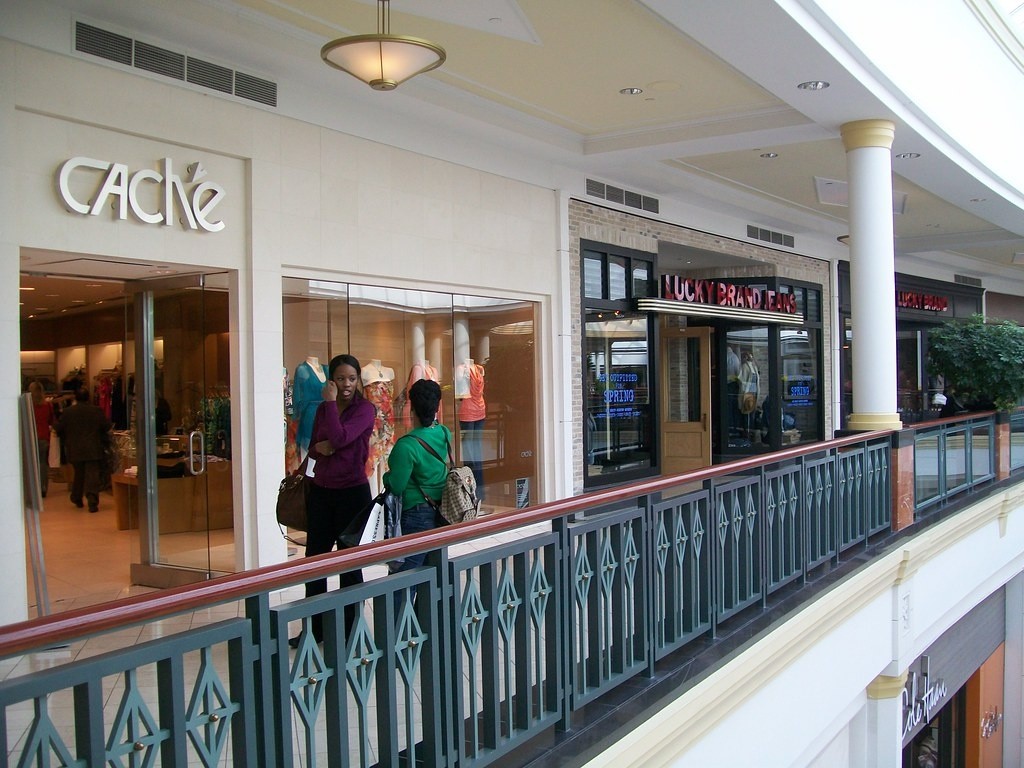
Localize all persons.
[293,356,486,515]
[383,379,452,667]
[727,347,761,429]
[28,382,53,498]
[276,354,377,648]
[53,388,113,513]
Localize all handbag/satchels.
[276,475,307,532]
[48,436,68,469]
[337,487,406,573]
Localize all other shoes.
[88,503,99,513]
[70,494,84,508]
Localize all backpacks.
[398,424,482,525]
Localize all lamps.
[321,0,447,92]
[837,235,849,244]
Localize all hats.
[742,392,756,414]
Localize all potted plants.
[926,311,1024,435]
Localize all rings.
[330,451,333,454]
